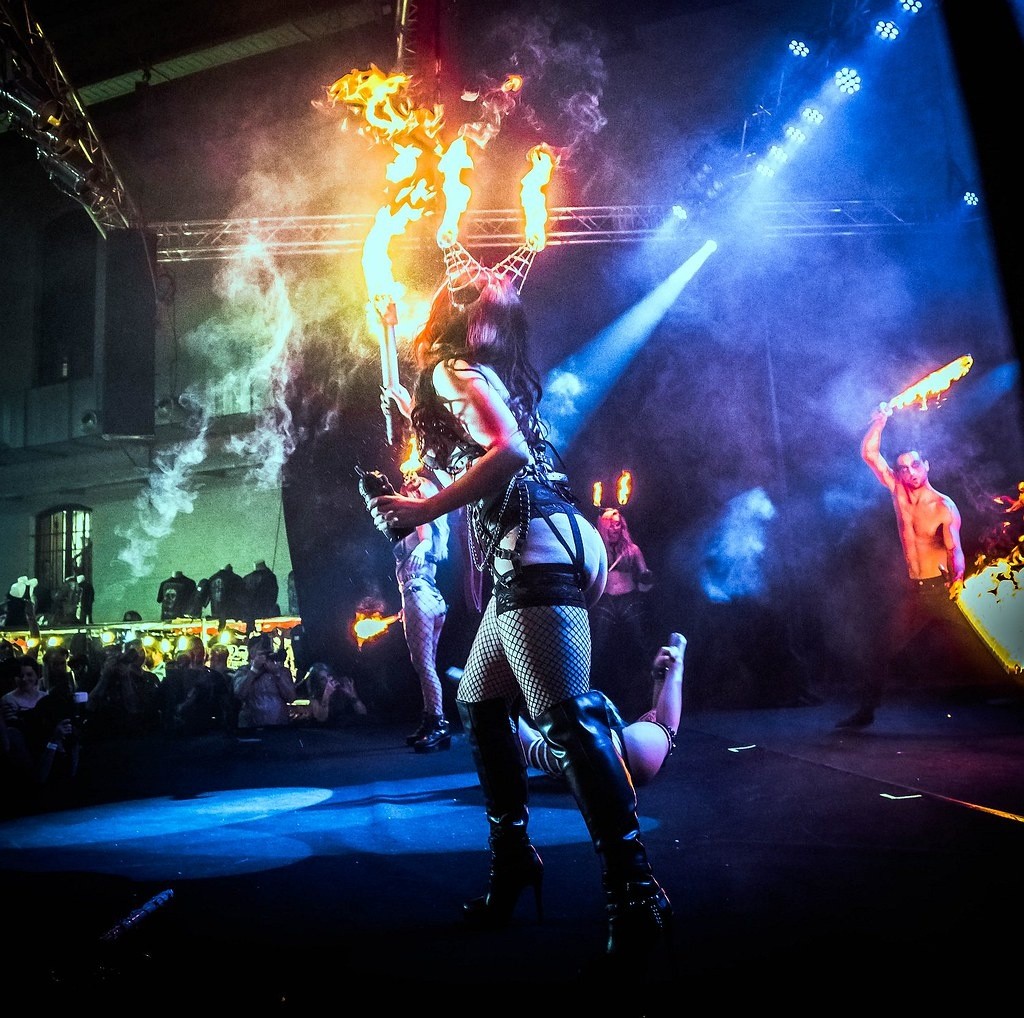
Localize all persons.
[0,550,299,621]
[232,633,298,732]
[831,407,1020,732]
[390,429,459,753]
[365,261,689,999]
[304,662,368,731]
[588,504,658,701]
[0,594,233,797]
[440,628,694,787]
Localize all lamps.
[0,75,65,130]
[35,141,100,195]
[8,106,86,156]
[686,142,735,200]
[50,171,102,207]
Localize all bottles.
[355,464,417,546]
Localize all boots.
[415,713,452,753]
[406,711,432,747]
[535,688,674,980]
[455,693,548,927]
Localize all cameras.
[257,649,288,670]
[110,647,139,677]
